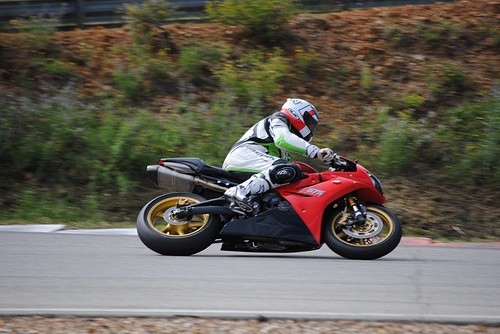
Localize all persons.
[222,98,335,215]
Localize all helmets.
[280,98,319,143]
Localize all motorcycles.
[136,152,403,260]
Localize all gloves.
[317,147,336,164]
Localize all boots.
[224,174,266,212]
